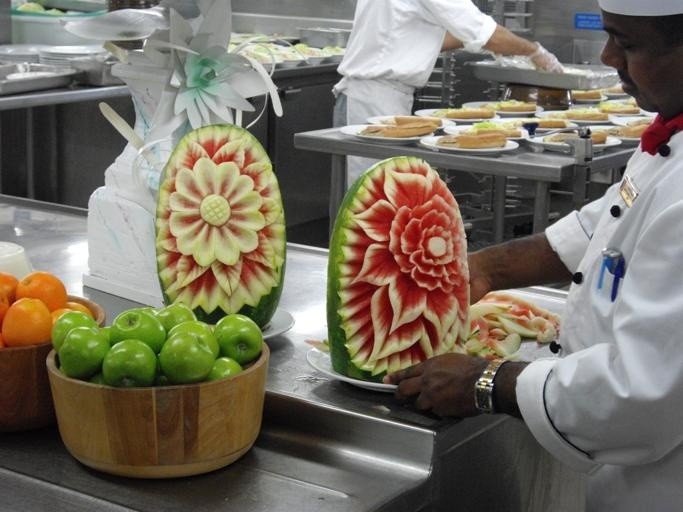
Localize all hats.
[598,1,682,18]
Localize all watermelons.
[327,157,471,384]
[155,124,288,329]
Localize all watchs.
[474,358,509,415]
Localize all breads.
[360,84,652,148]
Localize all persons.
[328,0,564,246]
[381,1,683,511]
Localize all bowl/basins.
[40,348,274,476]
[0,296,105,431]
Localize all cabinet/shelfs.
[230,84,335,230]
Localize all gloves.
[527,39,565,73]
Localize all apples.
[51,302,263,389]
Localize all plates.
[534,121,579,131]
[254,306,294,341]
[415,109,501,124]
[304,340,398,395]
[419,135,520,154]
[231,39,344,71]
[444,125,529,141]
[536,111,611,124]
[338,124,435,146]
[0,43,106,69]
[464,101,544,117]
[523,134,623,154]
[571,90,658,144]
[366,115,455,131]
